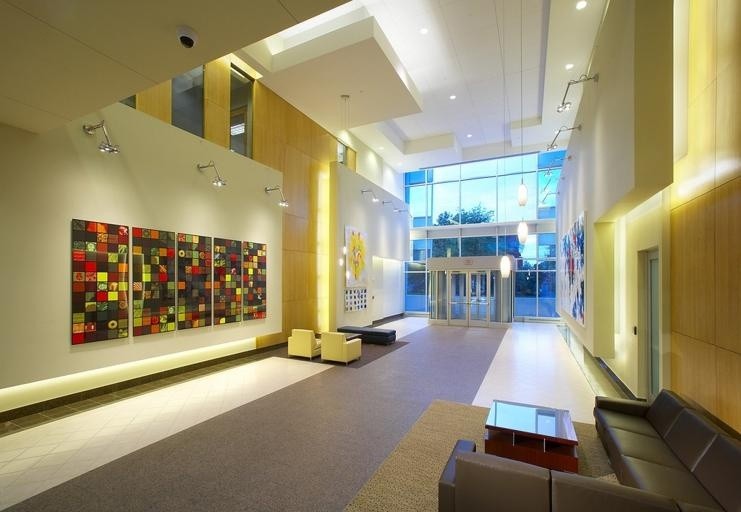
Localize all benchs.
[337,325,396,346]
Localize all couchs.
[592,389,741,512]
[438,440,725,512]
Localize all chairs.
[287,328,320,360]
[320,332,362,366]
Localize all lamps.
[264,183,290,208]
[492,0,598,278]
[197,160,226,187]
[83,119,121,155]
[361,189,412,219]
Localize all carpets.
[271,342,410,369]
[344,399,620,512]
